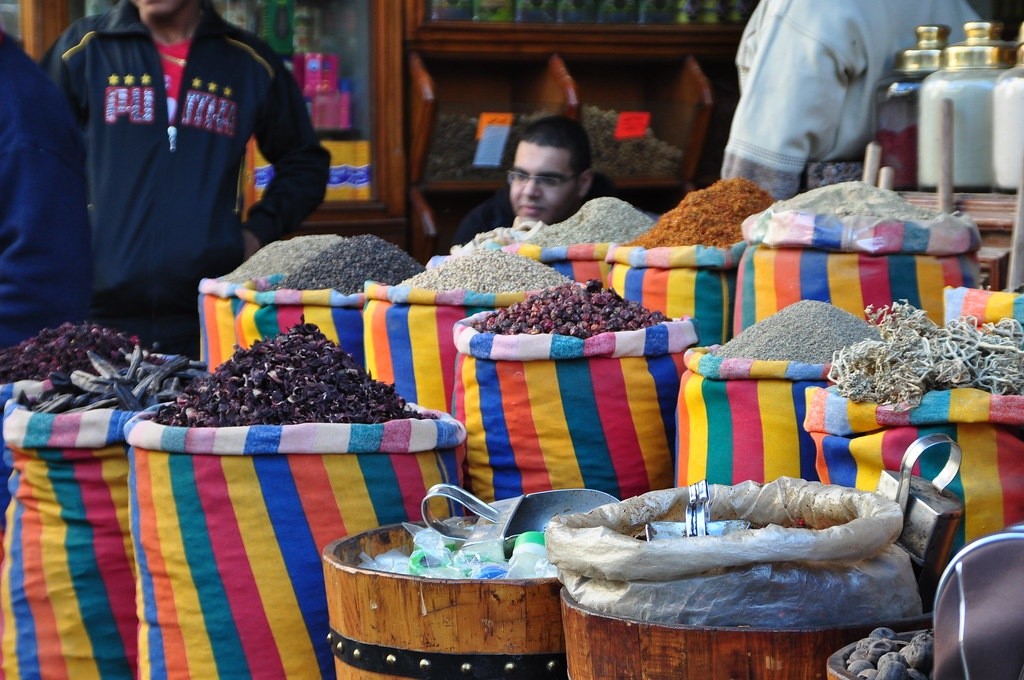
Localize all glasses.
[506,169,576,188]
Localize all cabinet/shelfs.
[19,0,407,251]
[407,0,745,266]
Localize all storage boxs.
[240,52,370,207]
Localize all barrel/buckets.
[321,518,567,680]
[558,586,934,680]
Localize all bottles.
[873,22,1024,195]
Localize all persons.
[721,0,984,202]
[448,115,626,256]
[0,25,92,351]
[38,0,331,362]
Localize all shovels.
[418,482,621,552]
[644,478,751,544]
[875,433,966,591]
